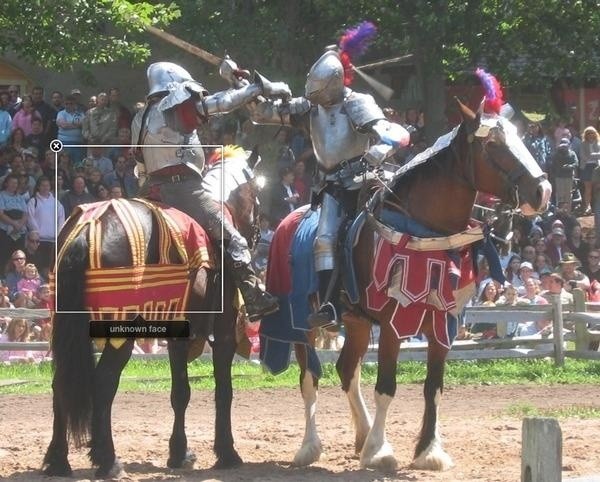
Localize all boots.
[306,269,343,333]
[237,261,280,318]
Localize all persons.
[219,45,411,333]
[0,84,600,363]
[130,62,293,322]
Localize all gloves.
[252,68,292,105]
[364,142,395,169]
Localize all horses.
[265,95,554,474]
[38,142,263,482]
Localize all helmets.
[303,49,346,107]
[144,61,210,96]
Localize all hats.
[558,251,581,264]
[71,88,80,95]
[550,227,563,236]
[551,219,564,228]
[518,261,533,271]
